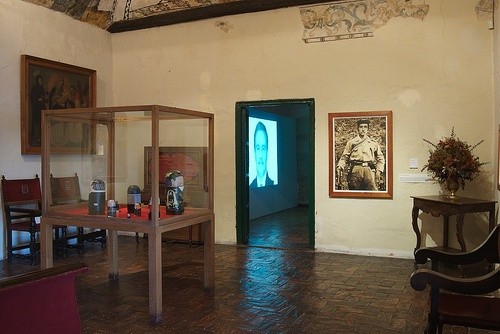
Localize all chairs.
[1,174,68,264]
[51,172,106,255]
[0,260,90,334]
[409,223,500,334]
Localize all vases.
[438,176,462,199]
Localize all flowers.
[420,126,489,190]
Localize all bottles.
[107,199,117,217]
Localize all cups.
[148,204,160,220]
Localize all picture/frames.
[328,111,394,200]
[20,53,97,154]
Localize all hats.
[356,120,370,124]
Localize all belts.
[353,161,373,166]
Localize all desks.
[410,195,498,264]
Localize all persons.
[336,120,385,191]
[249,122,276,189]
